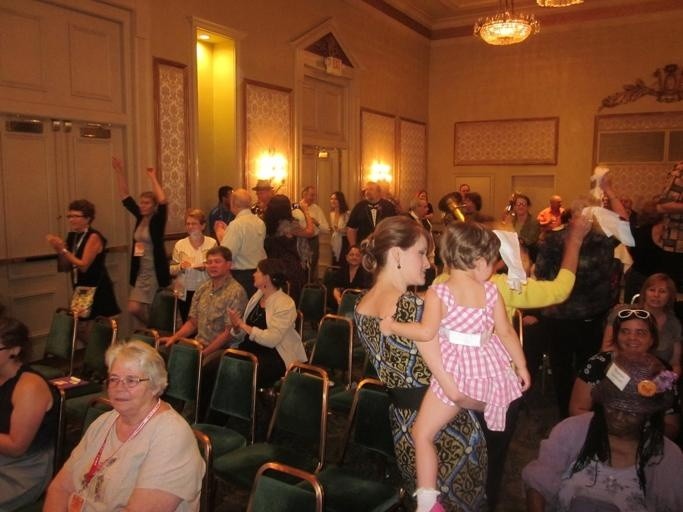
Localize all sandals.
[428,502,445,512]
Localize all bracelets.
[60,248,67,255]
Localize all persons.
[353,215,489,512]
[45,198,123,320]
[263,194,314,285]
[252,179,275,220]
[431,206,595,322]
[111,153,170,328]
[328,191,351,269]
[347,181,396,248]
[463,192,482,218]
[213,189,266,298]
[209,185,235,234]
[0,317,56,512]
[410,190,433,214]
[409,198,436,291]
[601,195,608,209]
[535,195,564,241]
[534,174,628,318]
[42,340,206,512]
[155,246,246,374]
[168,209,219,324]
[601,272,683,368]
[569,307,679,437]
[459,183,470,195]
[298,185,329,277]
[331,246,371,306]
[200,259,308,389]
[620,194,637,225]
[651,159,683,264]
[521,353,683,512]
[380,221,532,512]
[503,194,538,246]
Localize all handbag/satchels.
[70,280,97,319]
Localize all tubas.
[438,192,464,221]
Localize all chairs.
[164,337,203,427]
[324,265,347,293]
[245,464,322,511]
[273,314,352,399]
[64,327,157,421]
[329,354,379,408]
[295,379,410,511]
[336,287,373,376]
[213,364,329,493]
[192,349,257,458]
[189,431,211,512]
[29,307,77,382]
[297,281,325,324]
[50,316,117,465]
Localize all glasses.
[67,214,83,219]
[106,374,150,389]
[617,309,650,320]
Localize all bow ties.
[367,203,379,210]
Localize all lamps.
[536,0,584,8]
[474,1,539,46]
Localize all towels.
[493,227,527,291]
[586,205,636,249]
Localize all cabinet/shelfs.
[0,1,135,115]
[1,247,149,362]
[0,114,137,262]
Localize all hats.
[252,179,274,191]
[591,351,675,414]
